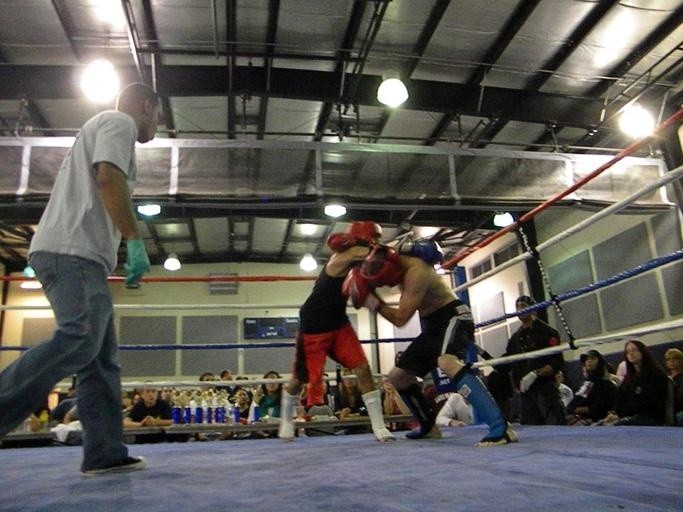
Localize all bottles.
[22,408,49,433]
[170,405,261,424]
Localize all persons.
[474,296,567,425]
[327,232,518,448]
[0,83,163,474]
[278,220,445,443]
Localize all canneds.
[207,407,213,423]
[232,407,240,422]
[183,406,190,423]
[254,407,260,420]
[195,407,203,423]
[215,406,224,423]
[171,406,180,424]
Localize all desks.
[4,415,418,443]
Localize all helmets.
[358,243,404,289]
[347,219,384,247]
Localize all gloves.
[340,265,383,314]
[518,367,540,394]
[121,238,151,289]
[324,231,359,253]
[397,237,445,264]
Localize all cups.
[294,406,304,418]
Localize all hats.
[579,349,599,363]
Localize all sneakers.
[78,454,146,477]
